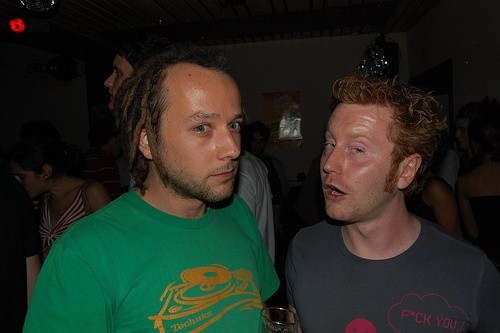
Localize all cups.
[260,307,297,333]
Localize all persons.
[285,72,288,76]
[0,41,297,332]
[285,72,495,333]
